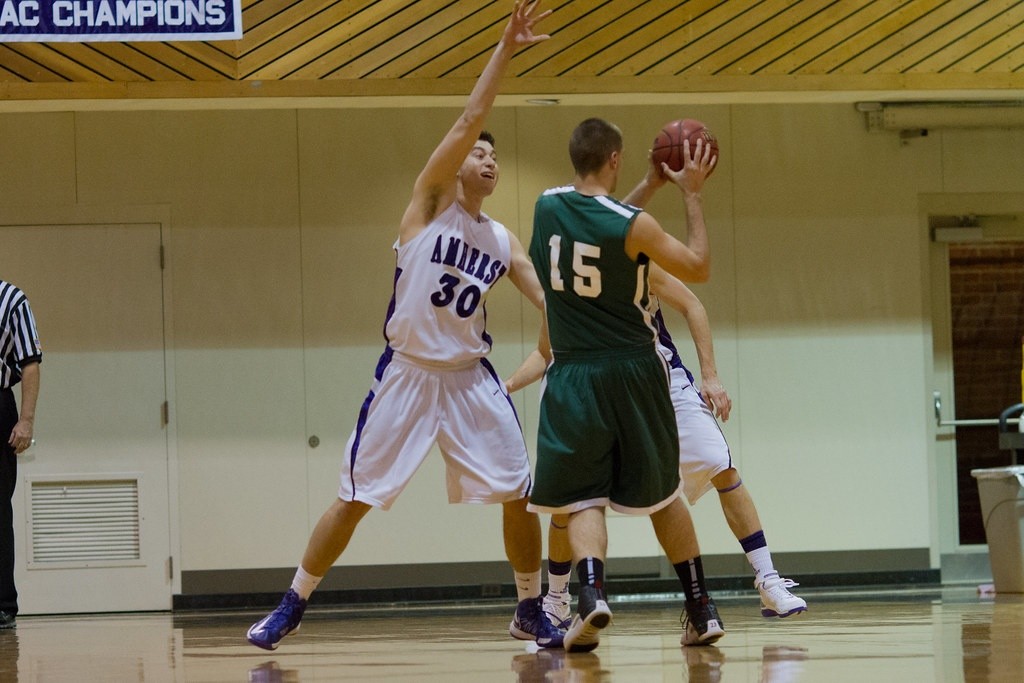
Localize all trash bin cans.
[970,465,1024,594]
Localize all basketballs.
[650,118,719,184]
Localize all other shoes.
[0,611,16,629]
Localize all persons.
[247,0,562,650]
[528,117,725,653]
[503,261,807,630]
[0,280,42,629]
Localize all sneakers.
[562,585,613,653]
[509,594,564,648]
[679,597,725,645]
[247,588,307,651]
[754,570,807,617]
[541,595,572,628]
